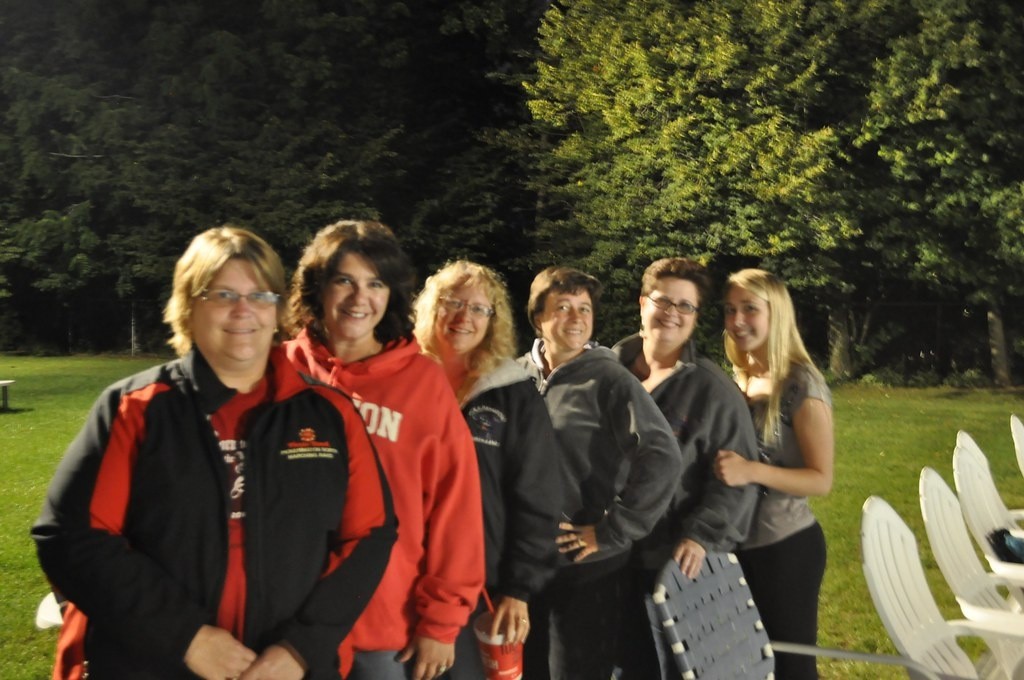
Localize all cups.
[474,608,523,680]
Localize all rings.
[438,666,446,671]
[519,618,526,623]
[578,539,582,547]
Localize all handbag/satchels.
[986,528,1024,564]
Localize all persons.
[29,227,399,680]
[409,261,564,680]
[713,269,835,679]
[280,220,486,680]
[610,256,759,680]
[515,265,682,680]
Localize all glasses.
[201,289,281,309]
[439,296,495,317]
[647,295,699,314]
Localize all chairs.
[1010,414,1024,477]
[953,445,1024,588]
[643,548,941,680]
[918,466,1024,670]
[956,430,1024,540]
[860,494,1024,680]
